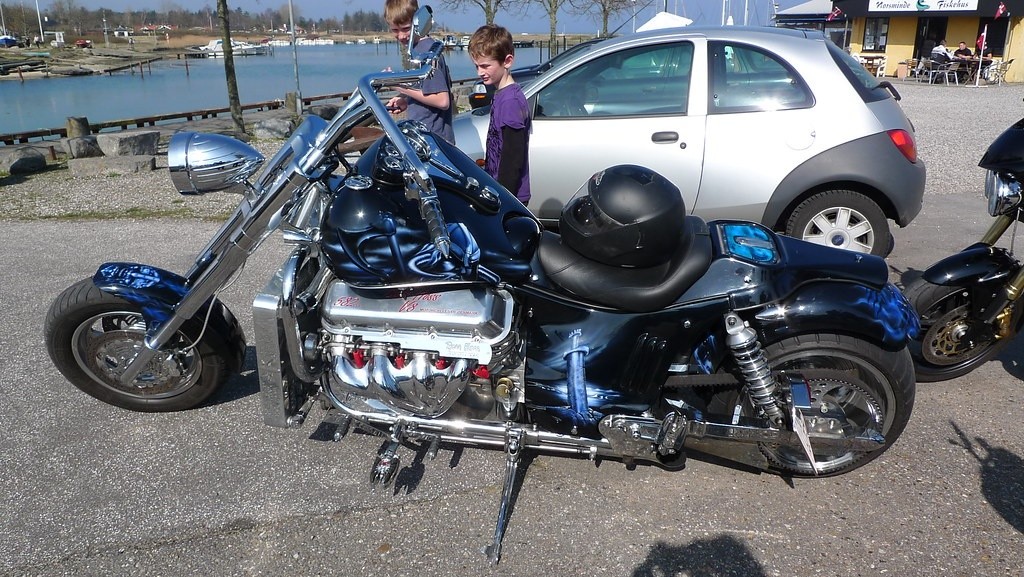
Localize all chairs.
[903,58,1015,87]
[852,54,887,77]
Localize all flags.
[827,8,841,22]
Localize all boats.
[199,37,270,56]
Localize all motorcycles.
[893,98,1023,383]
[44,2,922,567]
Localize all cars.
[452,24,926,262]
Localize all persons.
[384,1,456,146]
[467,24,532,209]
[916,33,992,83]
